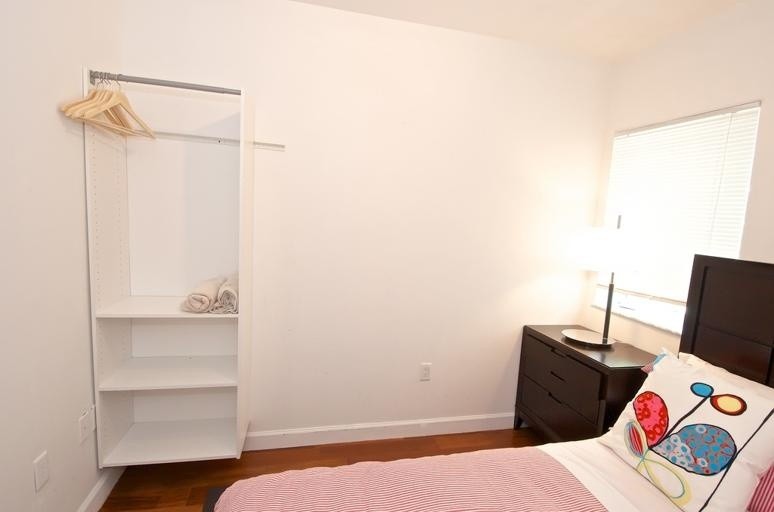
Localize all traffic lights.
[598,348,773,512]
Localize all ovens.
[212,252,773,512]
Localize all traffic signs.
[421,363,433,381]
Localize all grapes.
[514,324,660,441]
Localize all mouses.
[55,72,159,143]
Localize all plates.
[79,70,251,470]
